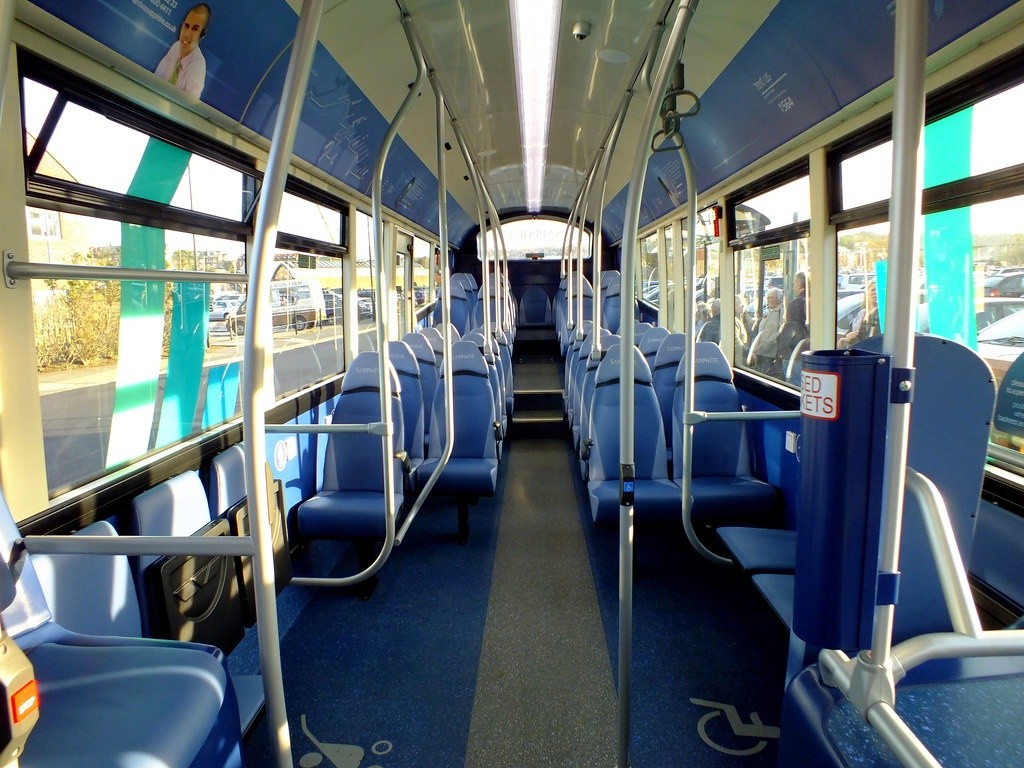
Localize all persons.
[694,272,881,387]
[154,3,211,99]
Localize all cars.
[209,285,426,327]
[634,261,1024,467]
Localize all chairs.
[0,272,1024,768]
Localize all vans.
[224,278,327,336]
